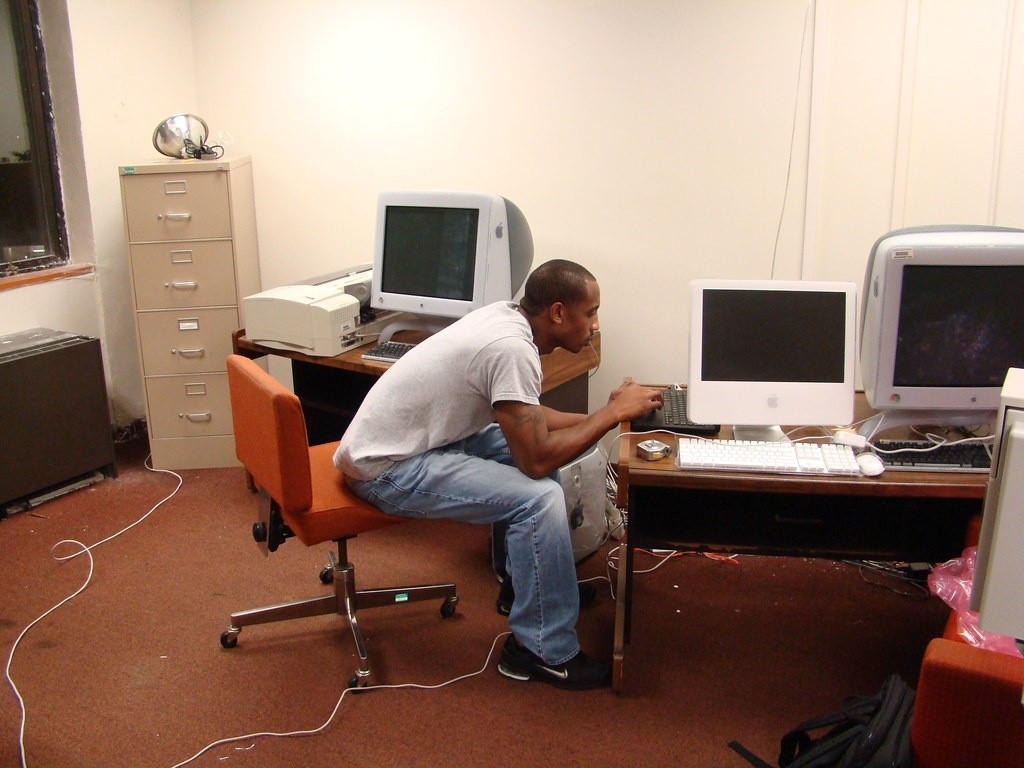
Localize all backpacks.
[728,674,916,768]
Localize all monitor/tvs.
[369,191,535,344]
[686,278,857,442]
[859,225,1024,438]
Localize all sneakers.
[497,633,612,690]
[497,572,596,615]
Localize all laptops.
[630,390,720,435]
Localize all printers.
[241,263,418,358]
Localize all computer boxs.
[967,366,1024,640]
[491,443,604,582]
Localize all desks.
[910,517,1024,768]
[613,384,991,692]
[232,327,602,449]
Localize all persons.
[331,256,664,691]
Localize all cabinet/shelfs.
[118,157,269,471]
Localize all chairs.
[221,354,461,693]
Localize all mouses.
[855,452,886,476]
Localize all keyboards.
[361,341,417,362]
[678,437,859,477]
[870,440,993,473]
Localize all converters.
[832,431,866,448]
